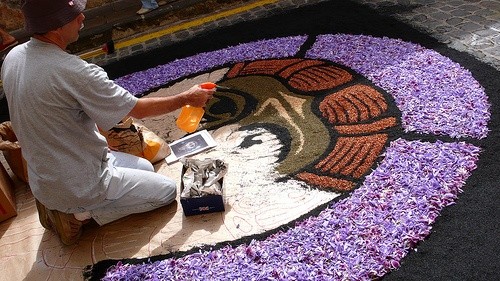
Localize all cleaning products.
[174,82,231,133]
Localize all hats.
[20,0,85,33]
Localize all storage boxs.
[0,165,18,222]
[180,164,226,217]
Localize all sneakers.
[47,207,83,245]
[34,198,53,230]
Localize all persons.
[0,0,214,246]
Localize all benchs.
[0,0,205,66]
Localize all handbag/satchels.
[105,115,170,163]
